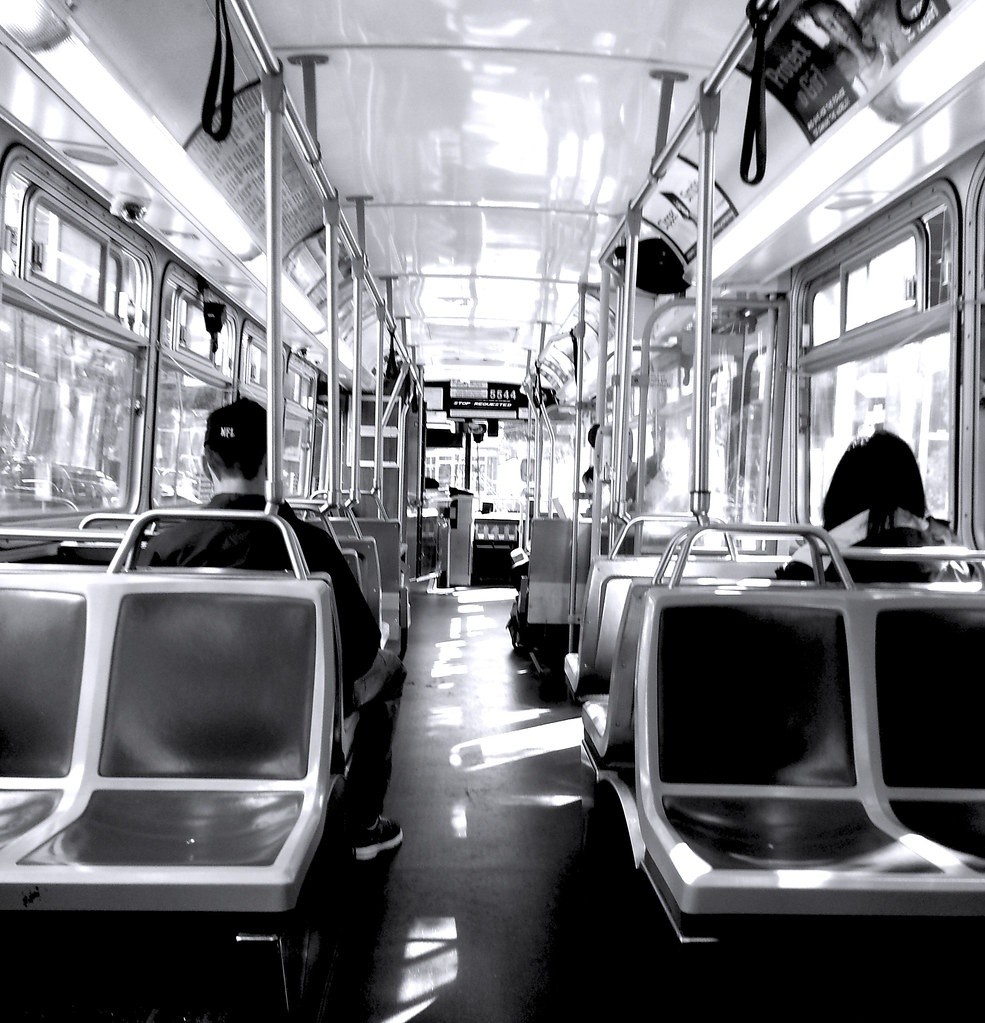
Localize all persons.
[775,429,977,585]
[580,424,665,519]
[135,397,402,860]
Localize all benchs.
[519,516,985,943]
[0,490,400,912]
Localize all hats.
[204,399,266,452]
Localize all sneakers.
[351,814,404,860]
